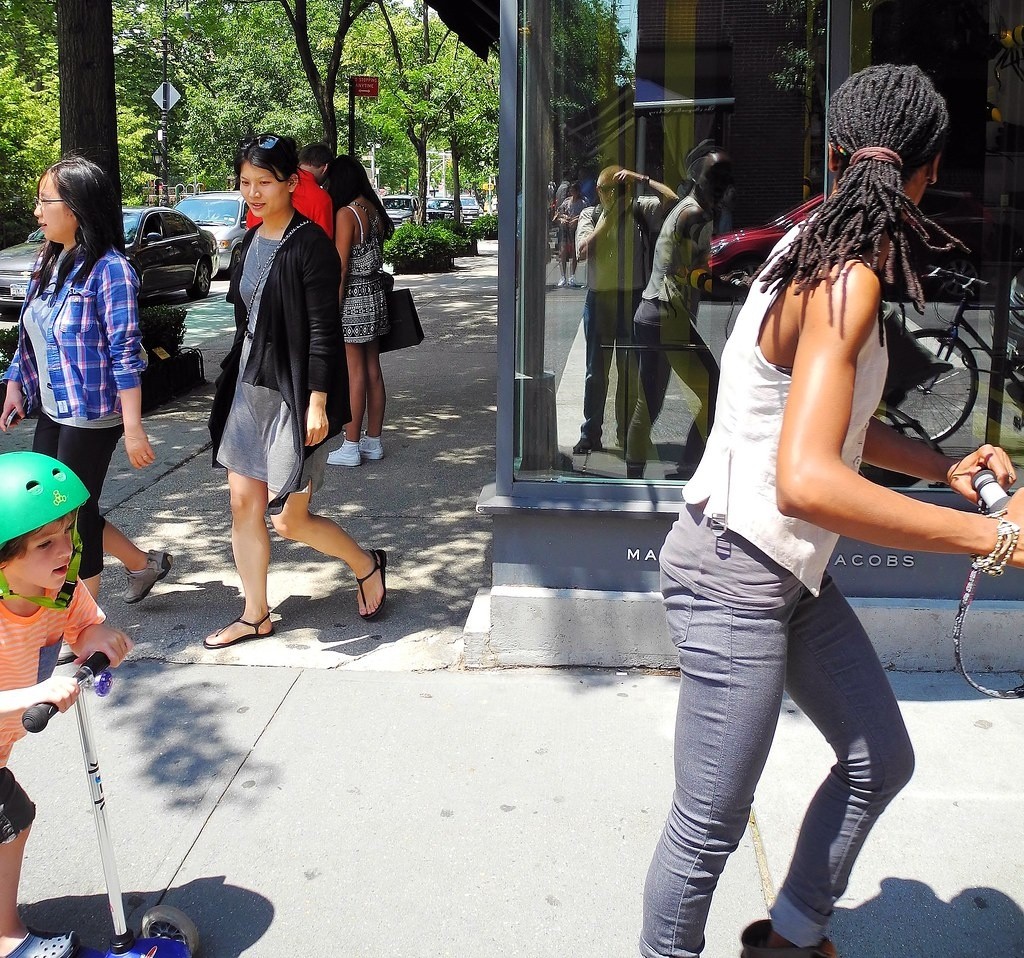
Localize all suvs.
[381,196,421,226]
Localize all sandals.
[203,611,275,650]
[355,549,387,620]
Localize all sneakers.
[57,640,79,666]
[122,549,173,604]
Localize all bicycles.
[886,264,1024,442]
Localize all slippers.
[0,926,81,958]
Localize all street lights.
[153,0,193,205]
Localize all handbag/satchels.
[375,288,426,354]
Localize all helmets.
[0,451,91,546]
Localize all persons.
[0,450,135,958]
[193,133,395,646]
[638,64,1024,958]
[0,158,172,668]
[550,139,732,481]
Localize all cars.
[0,206,221,307]
[173,192,249,272]
[426,198,464,224]
[461,197,483,221]
[706,185,1007,284]
[990,265,1024,370]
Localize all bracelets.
[641,175,651,187]
[971,516,1021,578]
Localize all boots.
[356,430,384,459]
[326,439,361,466]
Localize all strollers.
[973,468,1013,511]
[721,273,750,290]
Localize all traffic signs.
[352,75,379,98]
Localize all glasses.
[599,183,626,192]
[235,133,279,149]
[33,196,65,209]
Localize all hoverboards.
[19,656,199,958]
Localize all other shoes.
[567,277,576,286]
[572,436,603,454]
[614,436,625,448]
[557,276,567,287]
[739,919,839,958]
